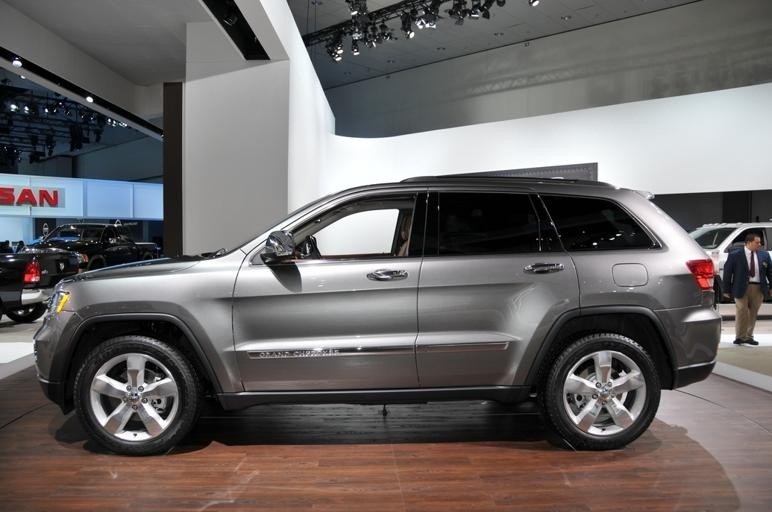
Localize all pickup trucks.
[0,218,161,327]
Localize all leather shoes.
[734,339,758,345]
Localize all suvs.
[31,172,728,460]
[687,217,772,302]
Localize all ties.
[750,251,755,277]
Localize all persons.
[722,235,771,346]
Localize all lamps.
[325,0,538,62]
[0,57,127,166]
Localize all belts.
[749,282,760,284]
